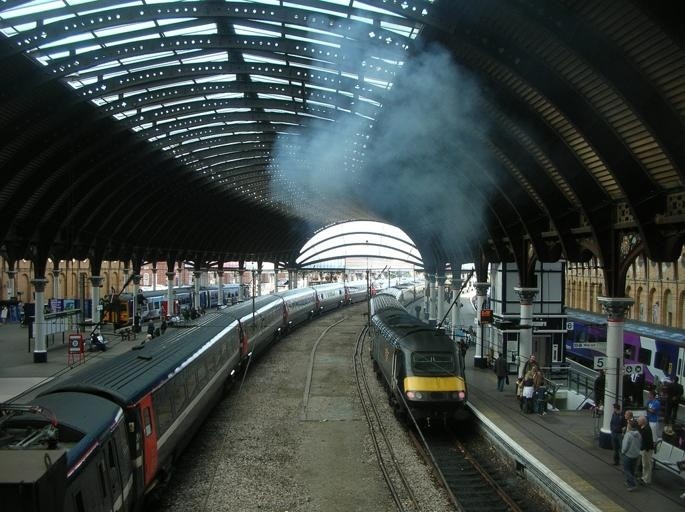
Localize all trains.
[564,305,685,408]
[367,272,469,432]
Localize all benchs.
[651,440,685,478]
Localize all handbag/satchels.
[506,375,509,385]
[523,386,534,399]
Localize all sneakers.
[623,480,650,492]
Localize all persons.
[646,390,660,448]
[663,376,683,423]
[494,352,508,392]
[515,355,547,416]
[147,305,205,339]
[456,339,468,372]
[134,311,141,327]
[414,304,422,319]
[10,301,25,323]
[91,333,104,345]
[594,369,605,418]
[467,325,473,335]
[610,403,653,491]
